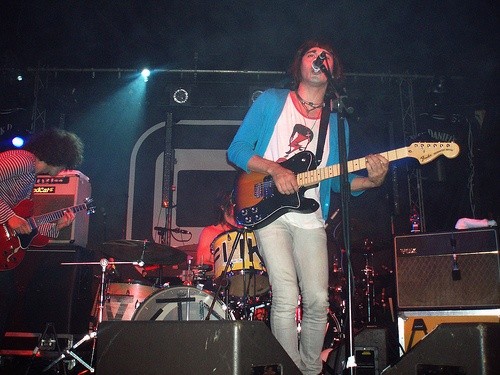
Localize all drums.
[86,278,160,336]
[130,286,236,320]
[209,226,270,298]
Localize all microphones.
[155,226,190,234]
[312,51,327,70]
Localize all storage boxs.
[397,309,500,357]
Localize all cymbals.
[162,244,202,278]
[94,238,188,265]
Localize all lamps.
[171,79,193,103]
[249,83,266,107]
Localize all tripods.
[38,261,143,375]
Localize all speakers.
[1,249,94,352]
[392,228,500,310]
[98,320,304,375]
[380,322,500,375]
[321,323,402,375]
[14,174,91,247]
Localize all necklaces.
[295,92,324,118]
[298,103,321,142]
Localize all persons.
[197,191,233,272]
[227,38,389,375]
[0,128,86,238]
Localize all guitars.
[231,141,461,233]
[0,195,96,273]
